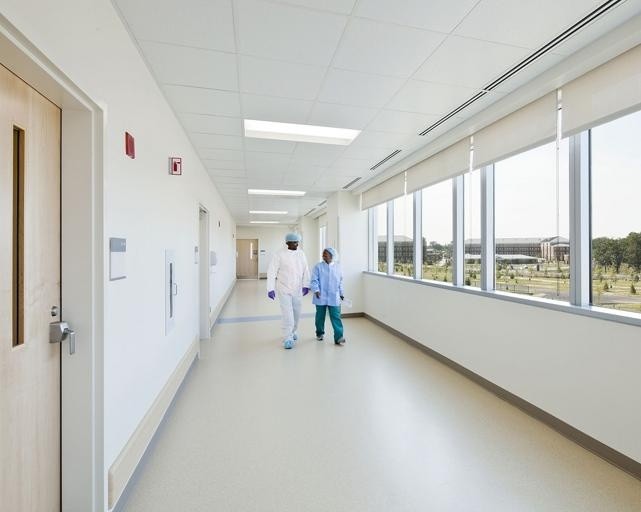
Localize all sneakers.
[316,333,325,341]
[284,339,294,350]
[334,337,347,345]
[292,332,299,340]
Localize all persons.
[266,232,312,350]
[311,247,348,345]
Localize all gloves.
[302,286,311,297]
[268,290,277,301]
